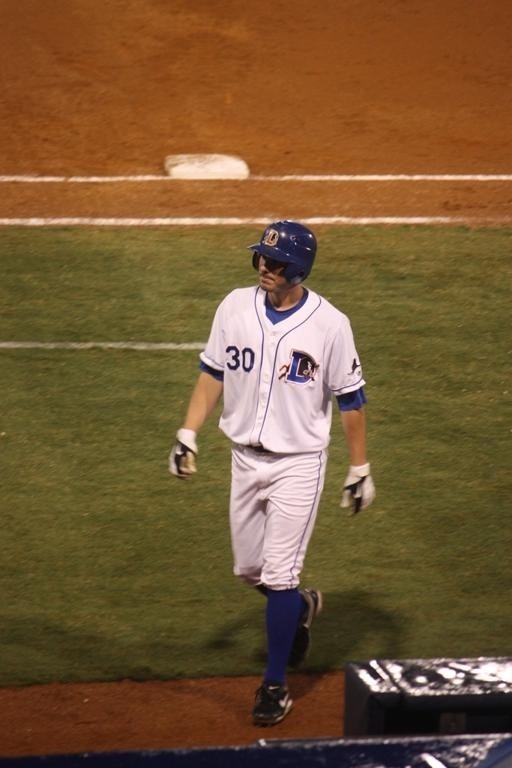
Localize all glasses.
[252,251,287,273]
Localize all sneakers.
[251,682,295,728]
[288,588,323,668]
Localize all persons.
[169,221,375,727]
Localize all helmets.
[245,220,318,278]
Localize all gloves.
[339,462,378,518]
[167,428,199,481]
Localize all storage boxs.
[342,655,511,735]
